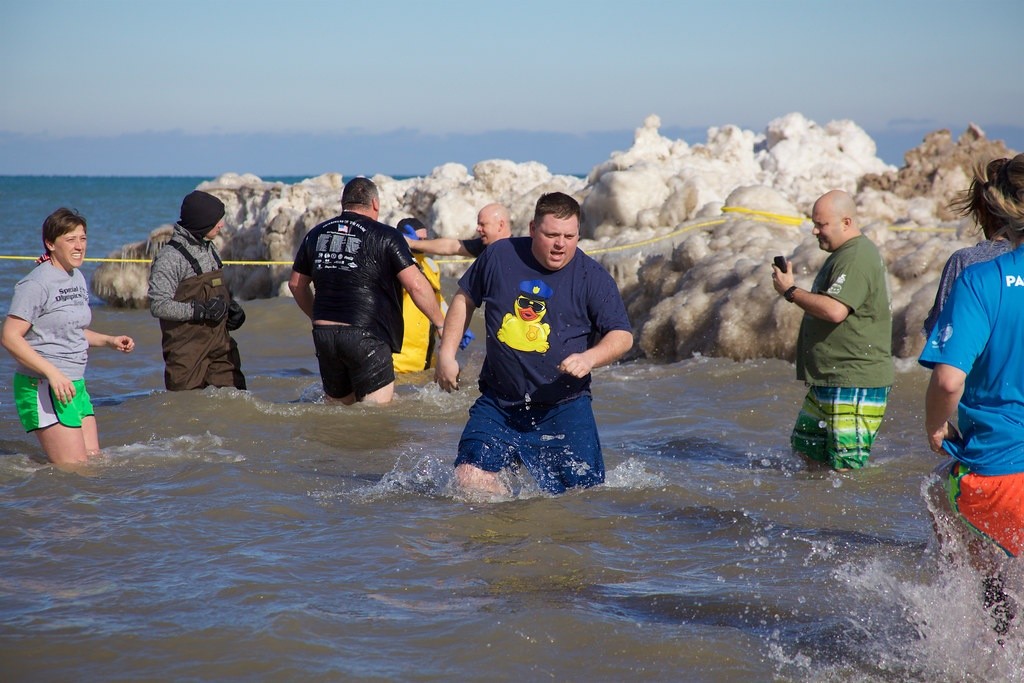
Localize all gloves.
[190,297,227,328]
[401,224,418,241]
[224,300,246,331]
[459,327,477,351]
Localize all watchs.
[784,285,797,303]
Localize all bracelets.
[435,324,446,330]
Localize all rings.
[772,272,776,279]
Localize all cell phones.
[774,256,787,273]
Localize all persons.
[923,156,1014,353]
[390,217,455,372]
[1,208,136,464]
[430,191,634,500]
[289,177,455,410]
[770,190,894,475]
[917,152,1023,654]
[398,204,514,260]
[147,189,247,392]
